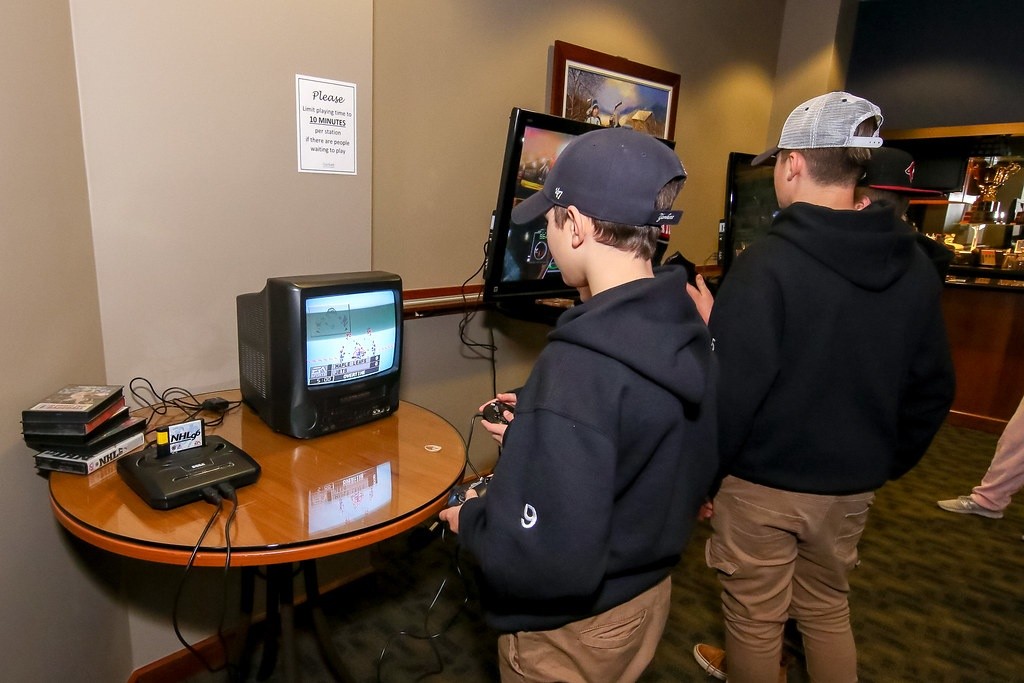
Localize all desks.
[47,383,468,683]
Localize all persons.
[855,146,955,279]
[937,391,1024,519]
[685,90,956,683]
[438,128,719,683]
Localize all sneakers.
[937,495,1004,519]
[693,643,788,682]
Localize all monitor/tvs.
[237,270,404,440]
[480,106,676,304]
[719,151,786,273]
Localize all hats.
[855,147,943,198]
[750,89,884,166]
[511,125,687,227]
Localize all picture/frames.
[550,40,681,141]
